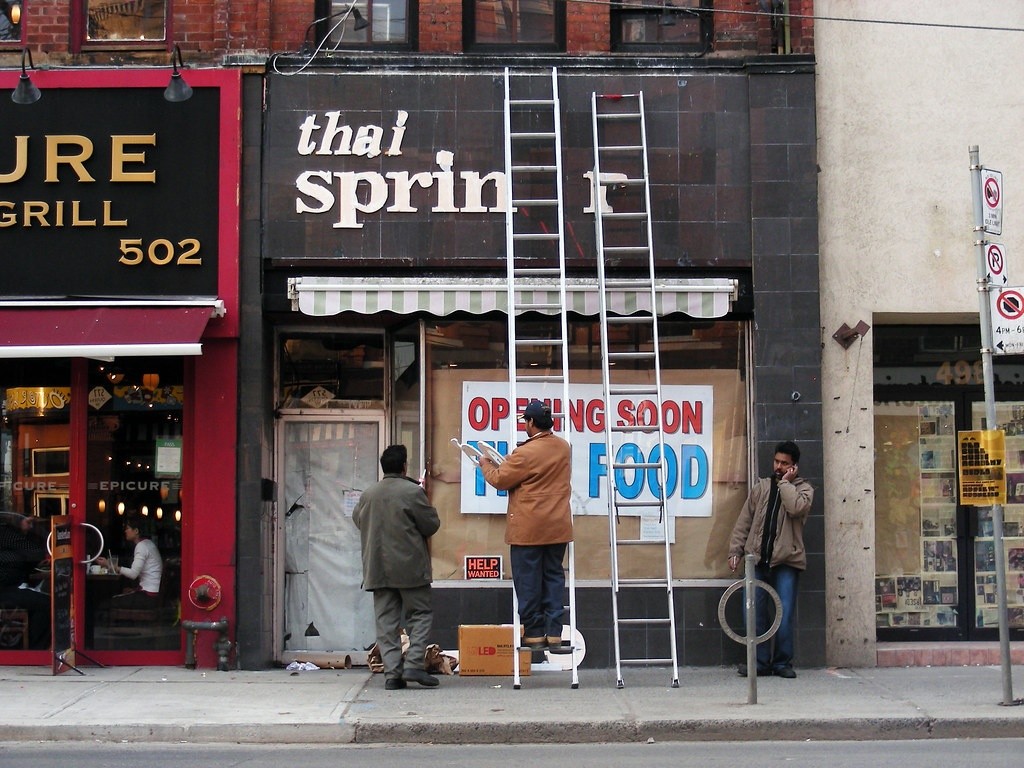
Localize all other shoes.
[773,667,796,678]
[520,636,545,649]
[402,668,440,687]
[384,678,408,690]
[545,635,561,649]
[738,666,764,677]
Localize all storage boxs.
[458,624,532,676]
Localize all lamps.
[12,47,43,105]
[164,42,194,103]
[658,2,712,58]
[11,0,21,25]
[298,6,371,55]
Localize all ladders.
[503,66,580,689]
[590,89,682,687]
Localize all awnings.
[0,300,227,359]
[287,277,739,319]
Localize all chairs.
[108,558,170,650]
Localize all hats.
[517,401,553,420]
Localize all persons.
[728,441,814,678]
[479,401,575,648]
[85,515,163,648]
[0,501,50,650]
[353,445,440,690]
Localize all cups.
[107,554,118,575]
[90,565,101,574]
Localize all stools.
[0,609,29,650]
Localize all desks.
[37,572,126,650]
